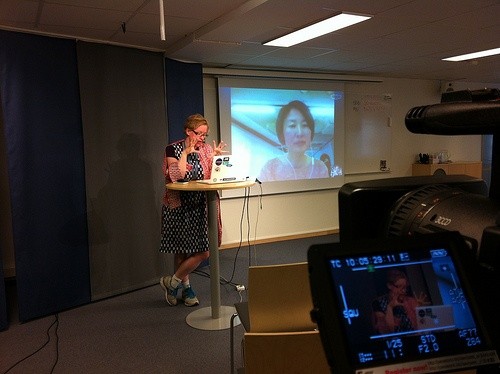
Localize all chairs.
[228,261,329,374]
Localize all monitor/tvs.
[307,230,500,374]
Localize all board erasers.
[381,160,386,166]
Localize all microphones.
[319,154,332,168]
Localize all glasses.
[189,128,209,137]
[390,282,408,289]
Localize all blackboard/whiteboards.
[344,91,394,176]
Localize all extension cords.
[236,284,245,291]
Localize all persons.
[260,101,328,182]
[160,115,229,306]
[371,269,429,335]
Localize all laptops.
[196,155,245,184]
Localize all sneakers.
[159,274,183,306]
[181,284,201,307]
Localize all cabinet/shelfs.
[410,161,482,178]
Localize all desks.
[165,176,256,331]
